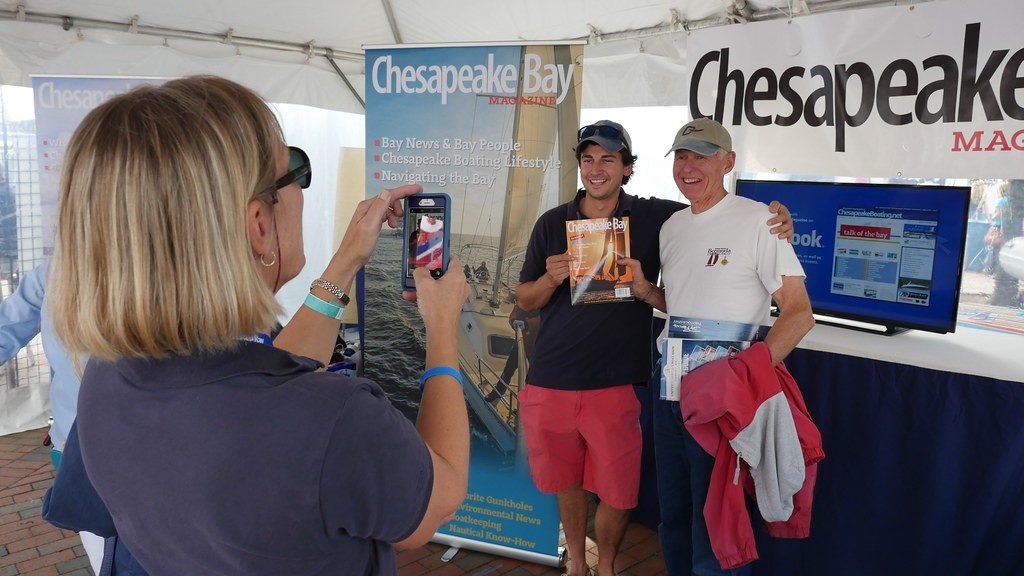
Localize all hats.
[664,118,732,156]
[576,120,632,155]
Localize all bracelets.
[419,366,464,391]
[310,279,350,305]
[640,282,653,302]
[304,293,345,320]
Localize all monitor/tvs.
[735,180,972,336]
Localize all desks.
[646,309,1024,576]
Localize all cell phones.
[401,192,451,292]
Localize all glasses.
[256,146,312,197]
[578,125,629,149]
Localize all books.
[659,338,761,402]
[566,217,634,305]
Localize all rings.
[388,206,394,211]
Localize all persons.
[968,181,1011,275]
[50,74,471,576]
[616,118,814,576]
[410,217,443,269]
[463,265,471,279]
[0,258,105,576]
[475,261,490,276]
[521,119,794,576]
